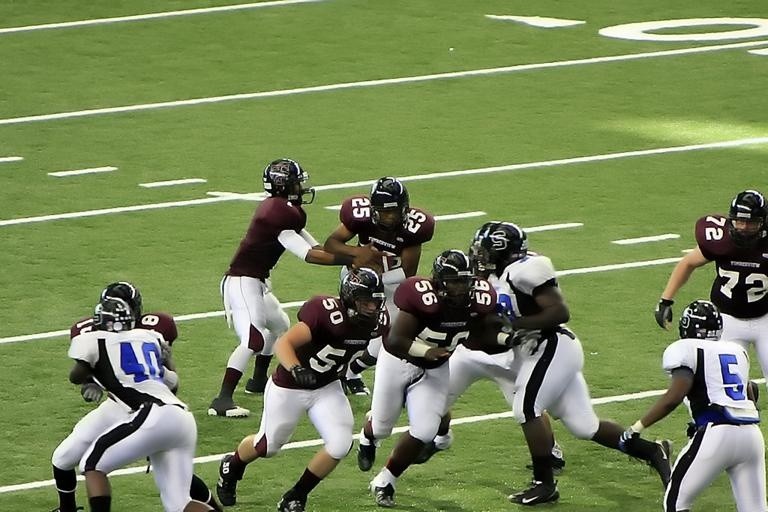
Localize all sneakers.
[509,440,564,505]
[217,455,245,506]
[359,428,452,507]
[277,495,307,512]
[648,439,673,491]
[245,377,269,394]
[346,359,370,396]
[207,398,250,417]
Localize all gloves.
[291,366,316,386]
[654,297,674,332]
[483,311,541,356]
[618,426,639,452]
[82,384,103,404]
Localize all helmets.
[263,158,314,197]
[679,299,723,339]
[93,282,141,331]
[728,189,768,239]
[370,177,409,227]
[341,267,387,323]
[433,222,527,307]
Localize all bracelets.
[495,331,510,346]
[630,419,645,433]
[407,340,431,358]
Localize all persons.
[616,298,768,511]
[652,189,767,385]
[213,221,673,512]
[50,282,224,511]
[335,251,353,266]
[205,156,380,417]
[322,175,436,395]
